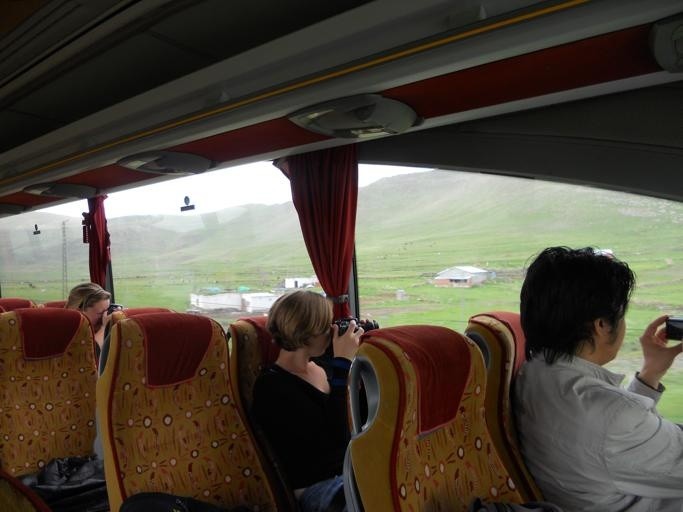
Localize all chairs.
[94,311,299,511]
[0,295,36,314]
[37,300,68,308]
[0,307,100,509]
[342,324,561,511]
[108,308,175,331]
[227,315,296,512]
[466,311,543,508]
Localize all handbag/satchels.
[18,456,109,512]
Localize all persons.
[62,282,130,365]
[250,291,365,511]
[509,242,682,512]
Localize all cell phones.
[665,318,683,341]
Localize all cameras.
[106,304,123,315]
[333,318,379,336]
